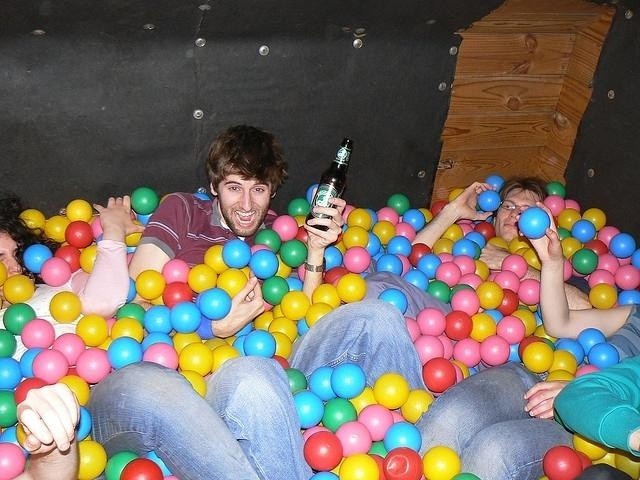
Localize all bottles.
[305,137,353,233]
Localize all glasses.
[501,199,529,217]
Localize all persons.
[222,335,237,347]
[414,202,640,480]
[1,194,313,479]
[11,382,82,479]
[205,337,228,351]
[433,239,454,255]
[128,126,432,480]
[172,332,202,356]
[362,177,593,323]
[552,353,639,480]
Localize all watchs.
[304,263,323,273]
